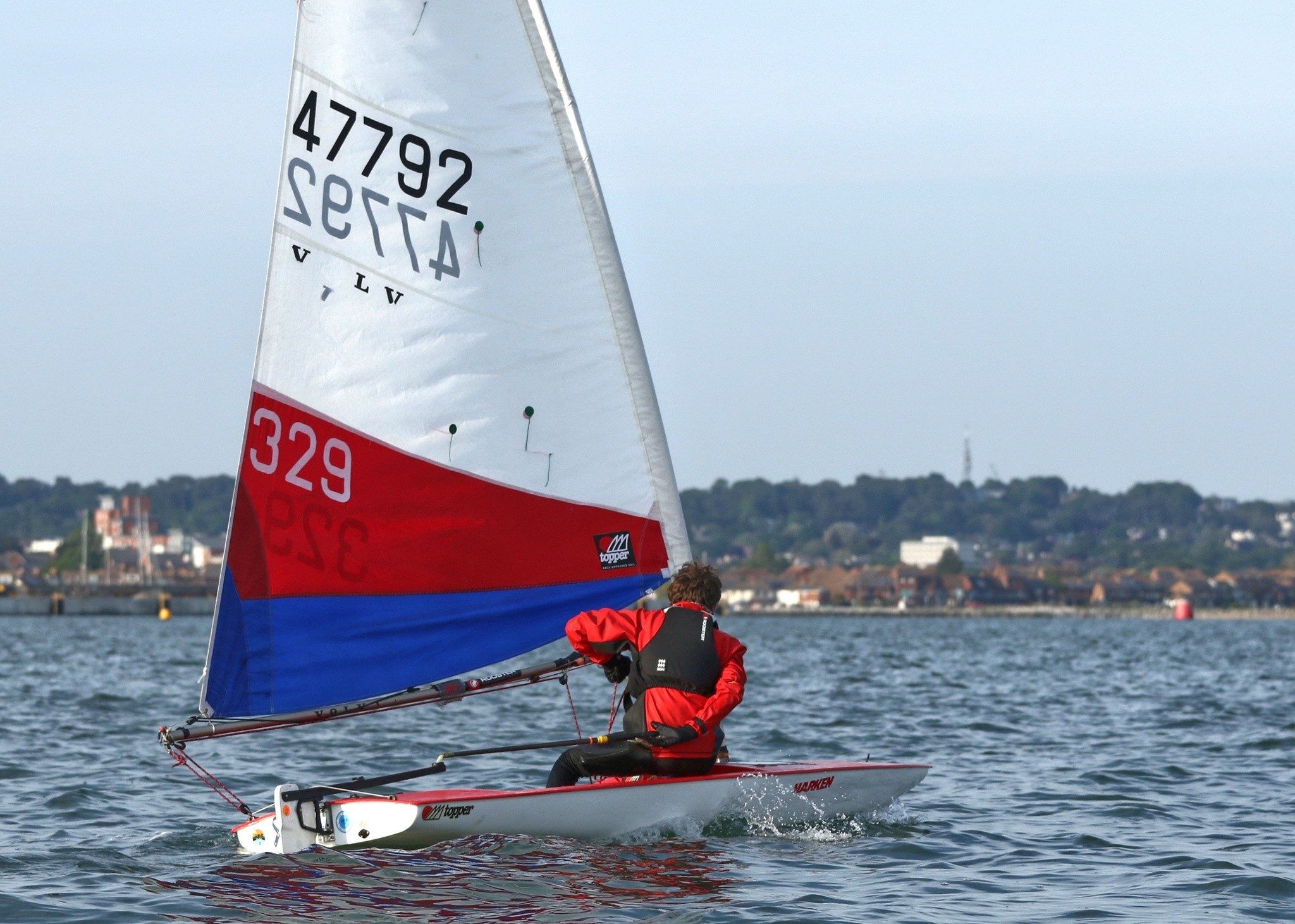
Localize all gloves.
[627,721,692,748]
[601,653,632,684]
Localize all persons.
[545,559,748,788]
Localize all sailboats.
[156,1,930,868]
